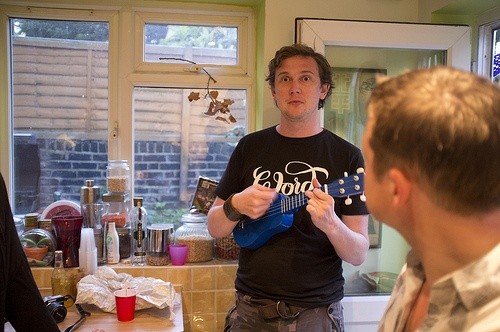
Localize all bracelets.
[223,194,244,222]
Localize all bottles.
[25,213,51,231]
[130,197,148,266]
[51,250,65,296]
[81,160,130,258]
[107,221,120,264]
[175,209,241,263]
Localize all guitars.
[232,166,366,250]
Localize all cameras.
[42,294,67,323]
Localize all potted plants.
[20,236,52,261]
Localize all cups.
[52,216,84,266]
[79,228,98,274]
[114,289,136,321]
[169,244,189,265]
[147,227,172,265]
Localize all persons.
[361,66,499,332]
[203,43,371,332]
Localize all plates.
[40,200,82,220]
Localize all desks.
[4,282,185,331]
